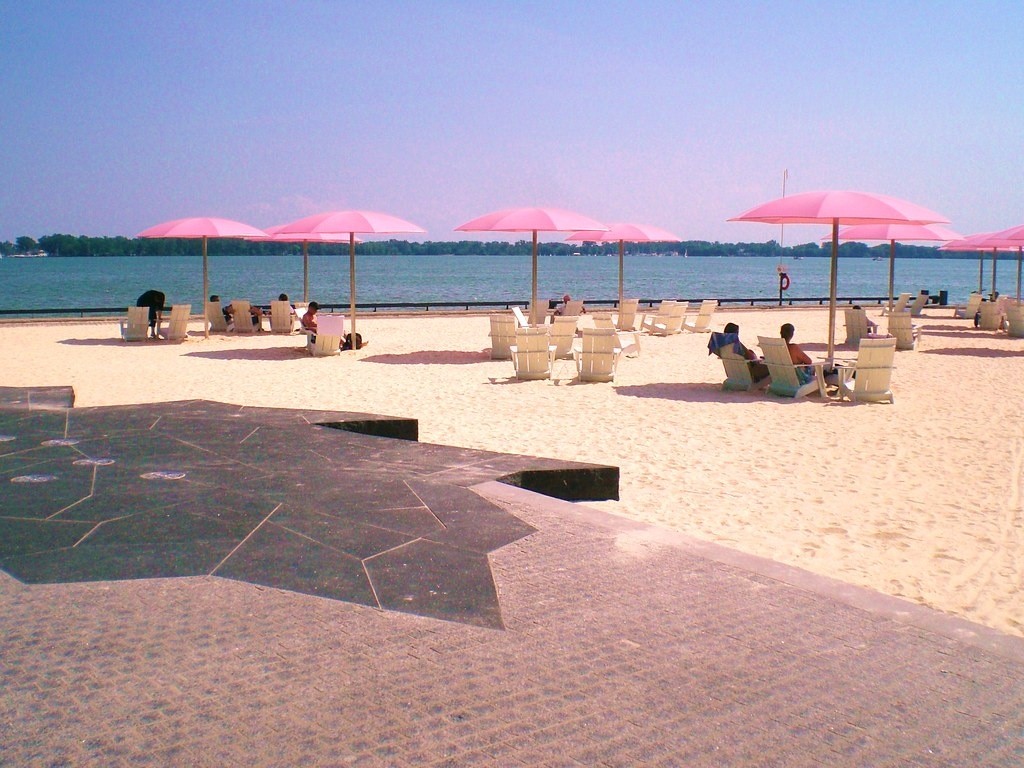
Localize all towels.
[707,331,745,358]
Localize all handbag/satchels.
[344,333,362,350]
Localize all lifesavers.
[781,275,790,290]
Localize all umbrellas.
[565,223,682,331]
[936,225,1024,302]
[821,225,965,311]
[273,210,427,350]
[135,216,269,340]
[453,207,611,327]
[245,224,362,303]
[725,190,952,358]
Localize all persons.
[974,298,986,327]
[780,323,815,375]
[137,289,165,337]
[242,299,264,331]
[302,301,349,345]
[724,322,769,379]
[209,294,233,322]
[853,305,875,333]
[268,294,296,329]
[554,295,586,332]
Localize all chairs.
[488,292,1024,404]
[122,300,345,356]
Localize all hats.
[309,301,321,309]
